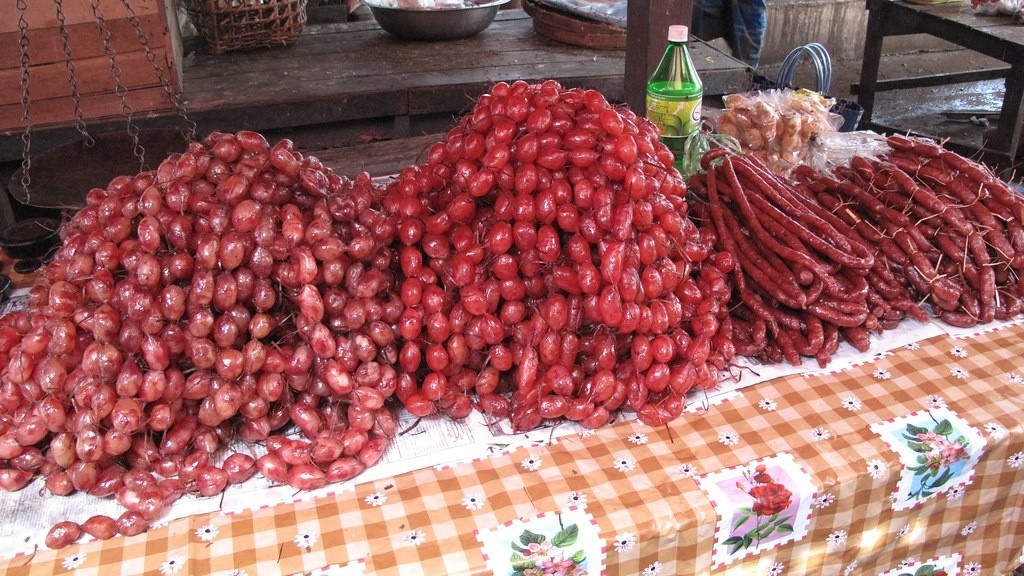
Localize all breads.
[715,91,837,168]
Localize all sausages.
[683,137,1024,368]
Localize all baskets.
[184,0,308,55]
[753,43,865,131]
[519,0,627,51]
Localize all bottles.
[644,25,703,181]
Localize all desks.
[859,0,1024,179]
[0,173,1024,576]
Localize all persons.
[691,0,767,71]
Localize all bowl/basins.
[360,0,513,41]
